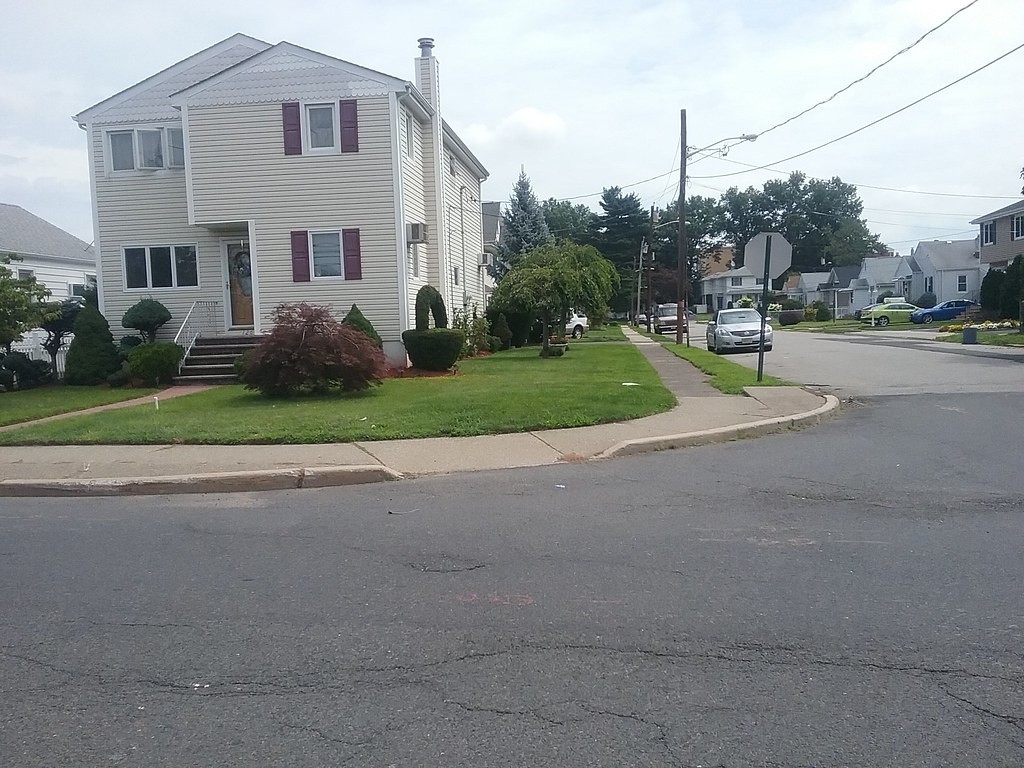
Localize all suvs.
[655,303,688,334]
[565,307,589,340]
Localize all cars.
[909,299,981,324]
[650,315,655,323]
[684,311,696,318]
[861,303,925,326]
[706,308,773,354]
[854,304,879,319]
[634,314,648,326]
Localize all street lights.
[647,220,691,332]
[677,134,758,344]
[637,268,654,325]
